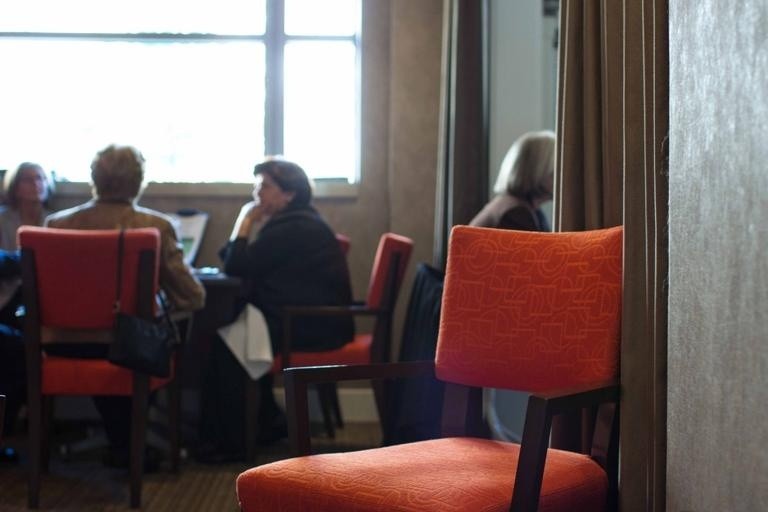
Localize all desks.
[191,267,240,471]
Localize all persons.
[0,161,58,253]
[44,144,209,474]
[461,129,560,235]
[183,157,357,466]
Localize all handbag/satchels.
[107,313,171,378]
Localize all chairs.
[270,233,415,460]
[17,225,182,510]
[236,225,623,509]
